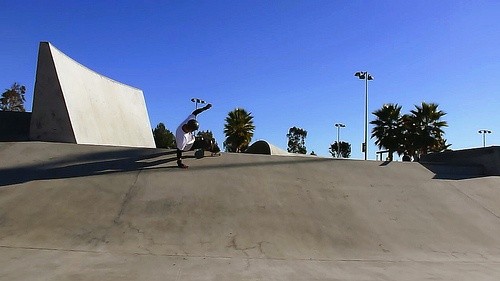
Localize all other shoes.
[207,138,222,154]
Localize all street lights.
[192,98,206,137]
[356,71,374,160]
[479,129,491,147]
[333,123,346,158]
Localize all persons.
[403,152,411,161]
[175,104,213,167]
